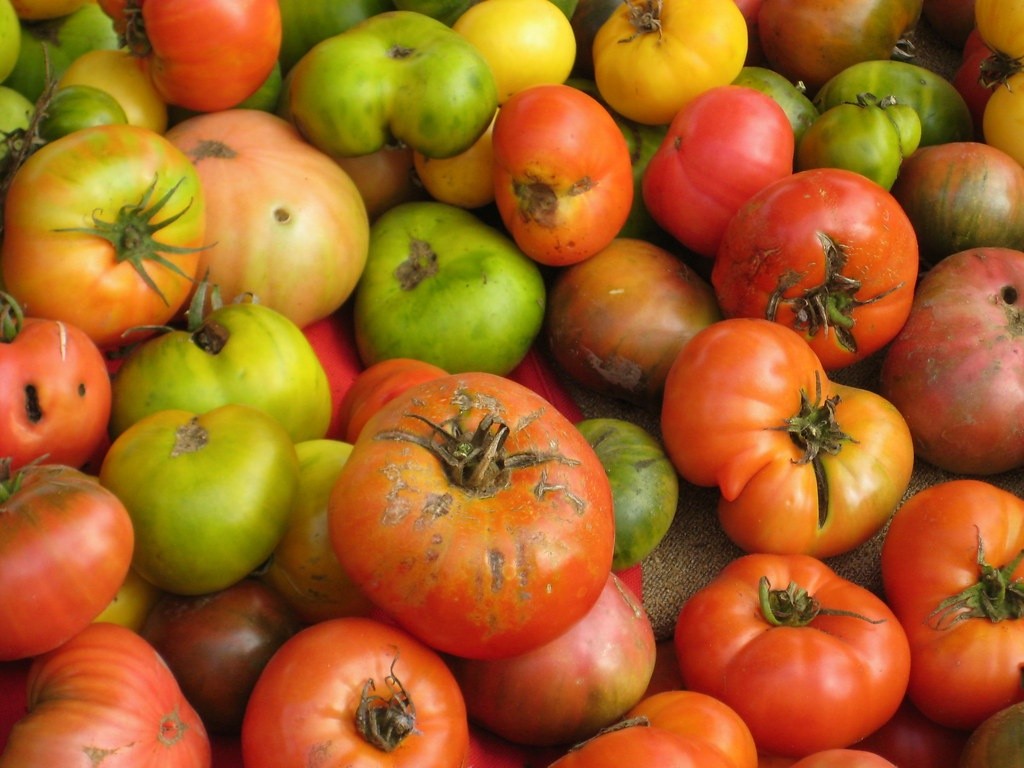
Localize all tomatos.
[0,0,1024,768]
